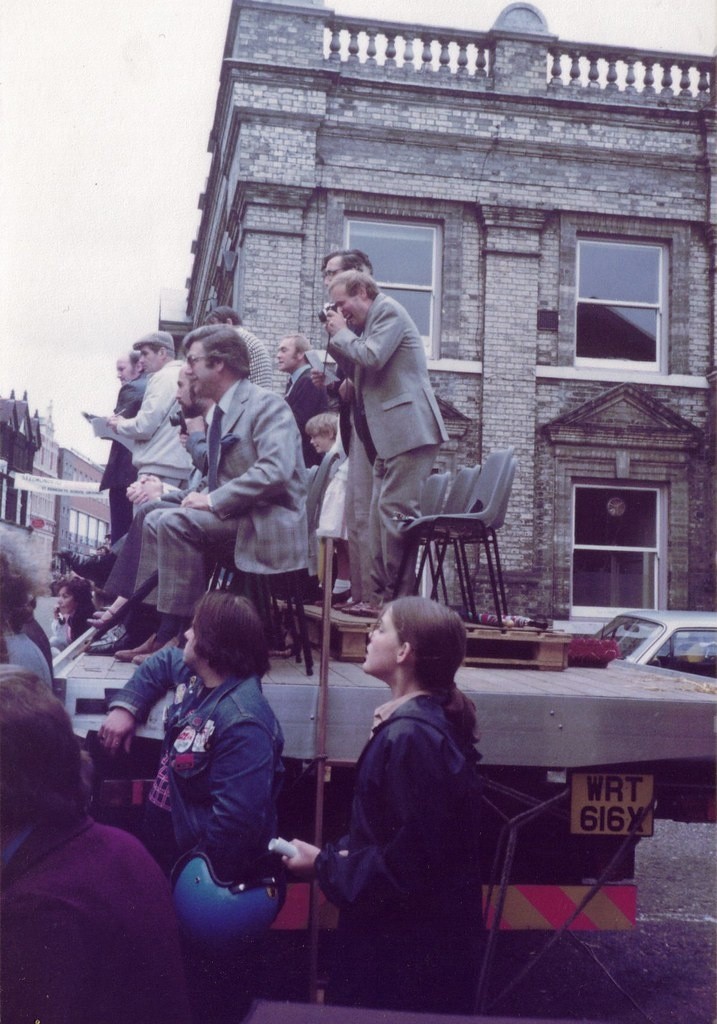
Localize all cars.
[591,609,717,678]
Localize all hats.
[133,332,175,352]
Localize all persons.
[0,531,54,697]
[97,592,284,1024]
[45,531,112,659]
[282,595,484,1015]
[278,250,449,616]
[87,305,309,664]
[0,662,196,1023]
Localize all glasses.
[186,352,206,367]
[321,268,342,281]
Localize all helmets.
[169,852,287,962]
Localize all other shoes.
[100,607,110,612]
[93,611,104,619]
[86,609,116,630]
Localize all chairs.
[208,449,341,676]
[397,445,521,628]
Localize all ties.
[209,405,224,493]
[285,376,293,394]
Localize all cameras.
[318,303,337,323]
[170,410,187,434]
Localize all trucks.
[40,600,717,942]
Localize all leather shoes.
[285,585,383,618]
[88,624,127,654]
[115,632,163,662]
[58,547,111,589]
[133,654,150,665]
